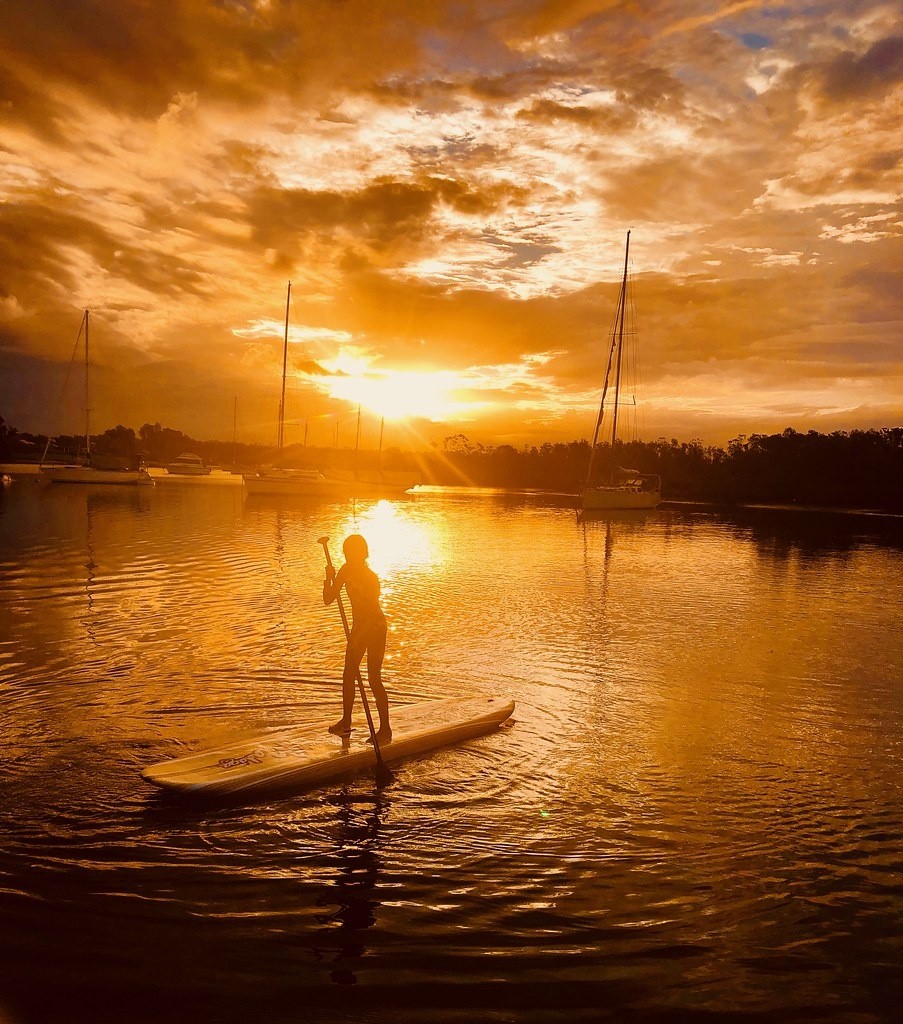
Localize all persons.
[323,534,392,743]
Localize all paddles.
[316,535,394,788]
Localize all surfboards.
[136,691,519,803]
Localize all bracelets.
[324,580,331,586]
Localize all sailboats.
[40,309,147,483]
[581,229,662,509]
[163,281,422,498]
